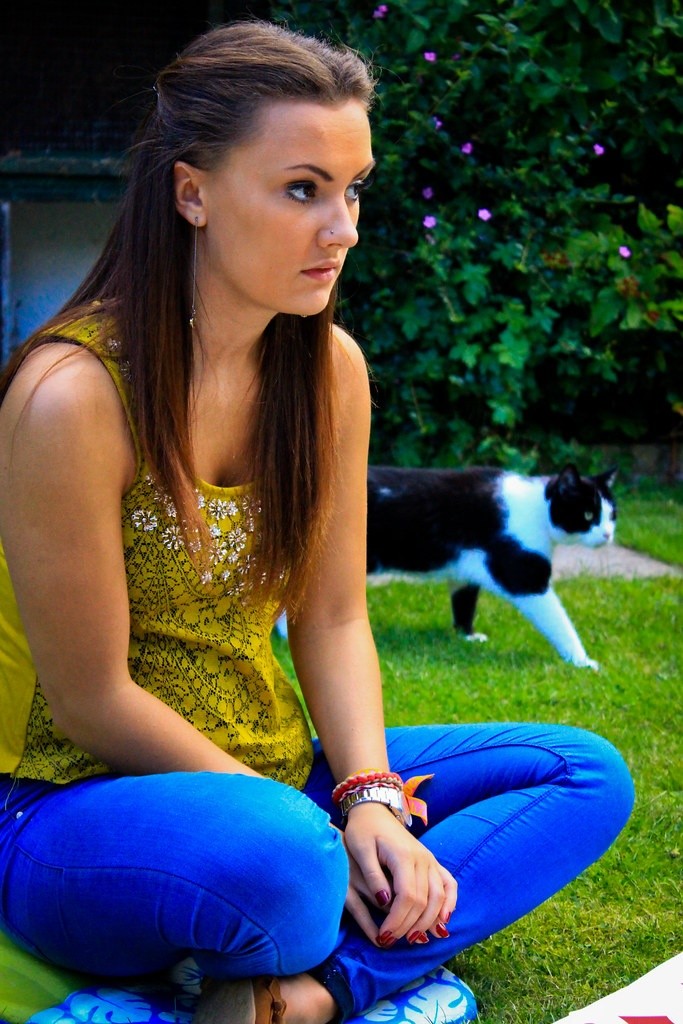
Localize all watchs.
[339,787,413,828]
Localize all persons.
[0,23,632,1024]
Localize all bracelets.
[331,766,436,826]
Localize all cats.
[276,462,618,672]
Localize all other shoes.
[191,973,288,1024]
